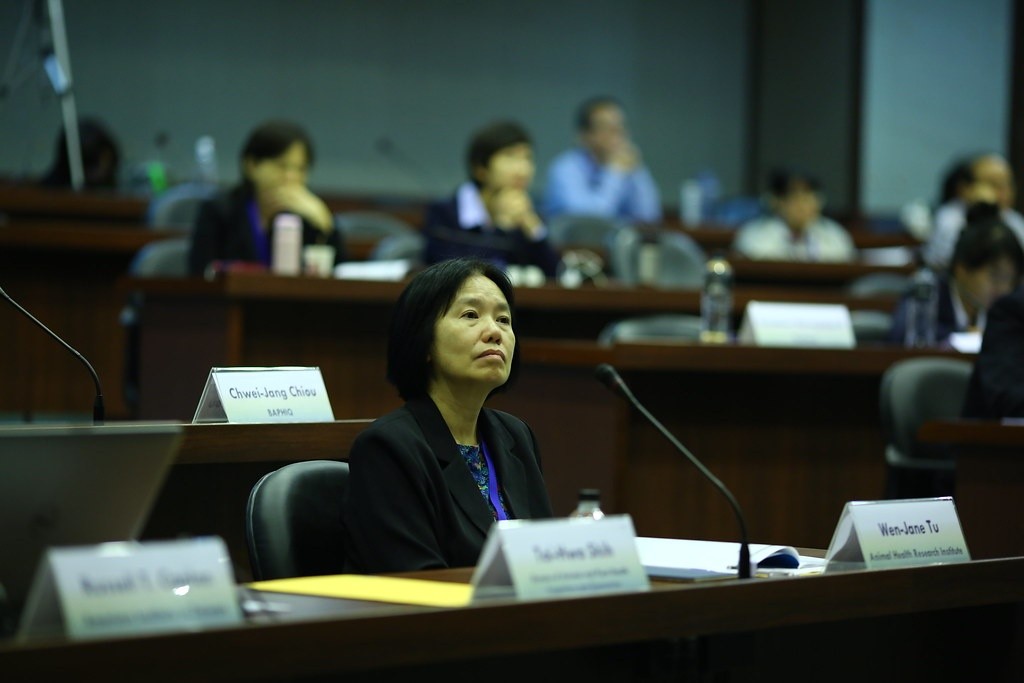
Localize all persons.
[540,96,660,223]
[423,120,567,277]
[893,147,1024,420]
[187,116,348,276]
[349,259,551,575]
[45,113,119,188]
[735,170,857,264]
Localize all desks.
[0,185,1024,683]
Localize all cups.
[301,245,336,280]
[272,212,301,278]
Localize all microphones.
[593,362,752,581]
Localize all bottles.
[568,488,603,518]
[902,269,939,349]
[700,259,733,347]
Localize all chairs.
[611,225,714,289]
[548,215,623,247]
[373,234,417,274]
[605,312,732,346]
[150,185,215,233]
[132,243,193,277]
[339,212,417,241]
[884,358,995,501]
[848,273,908,293]
[246,459,353,580]
[849,309,893,346]
